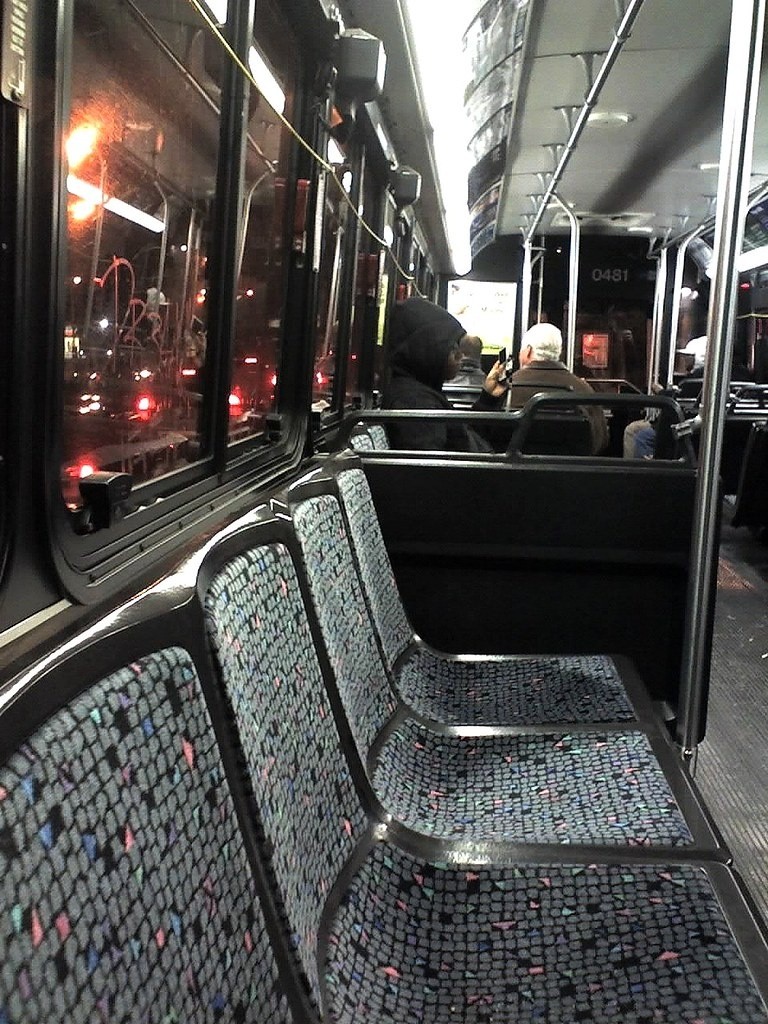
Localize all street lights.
[65,116,106,340]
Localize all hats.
[676,336,698,355]
[159,291,173,306]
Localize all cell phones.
[498,347,507,381]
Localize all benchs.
[1,376,768,1024]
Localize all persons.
[443,335,487,385]
[503,323,609,457]
[380,298,510,453]
[623,335,709,459]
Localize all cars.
[60,350,357,511]
